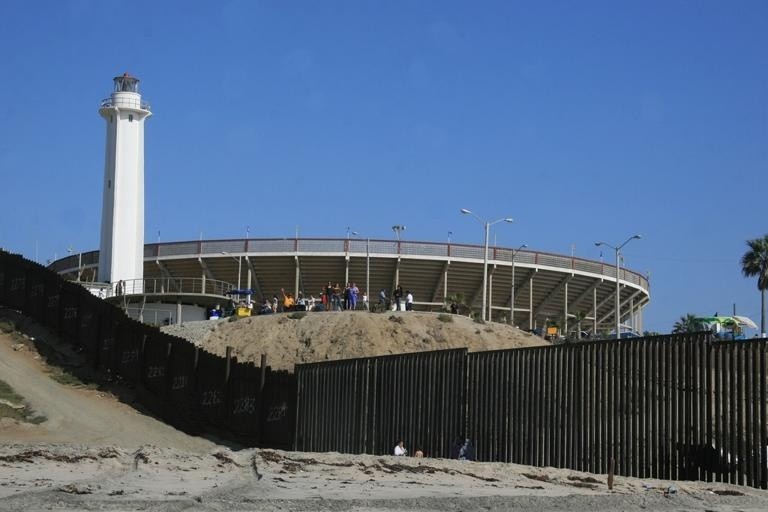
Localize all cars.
[525,329,600,343]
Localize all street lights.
[461,209,513,321]
[352,232,369,310]
[595,235,642,339]
[222,252,241,301]
[511,245,527,324]
[67,250,81,280]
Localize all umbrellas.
[223,287,257,295]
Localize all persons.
[393,441,407,455]
[262,278,359,314]
[362,292,368,310]
[228,299,235,315]
[393,285,402,311]
[405,290,413,311]
[380,288,387,309]
[451,301,459,314]
[415,448,423,456]
[114,280,122,296]
[98,288,104,299]
